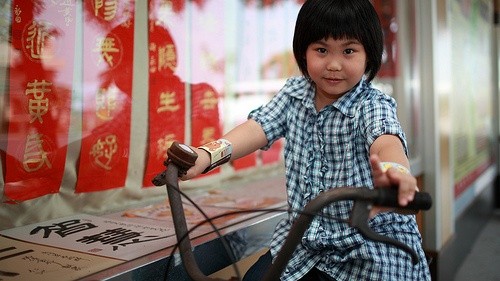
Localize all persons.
[180,0,432,281]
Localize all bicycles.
[150,141,431,281]
